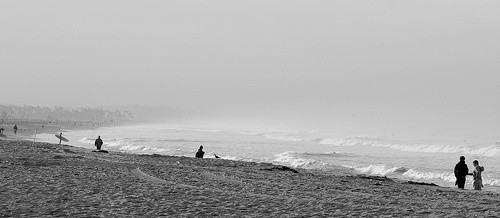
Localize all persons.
[195,146,204,158]
[95,136,103,150]
[455,156,468,189]
[468,160,482,190]
[14,125,17,134]
